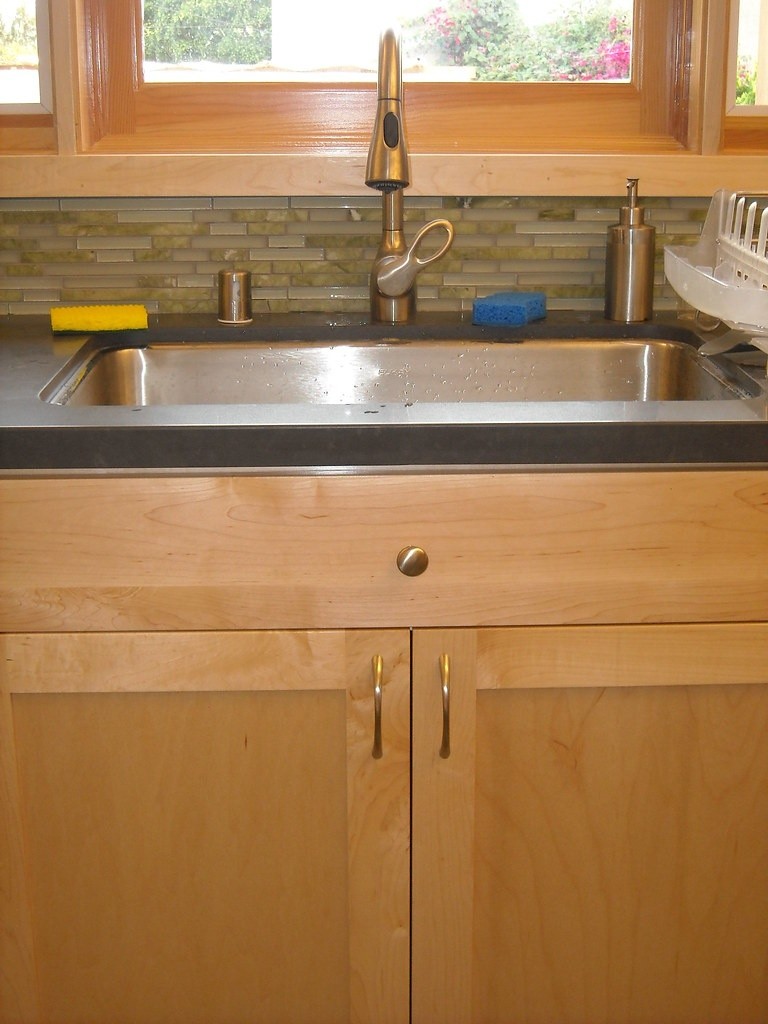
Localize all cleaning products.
[604,177,655,322]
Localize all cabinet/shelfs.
[0,478,768,1024]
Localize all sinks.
[39,334,766,406]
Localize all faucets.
[363,21,453,324]
